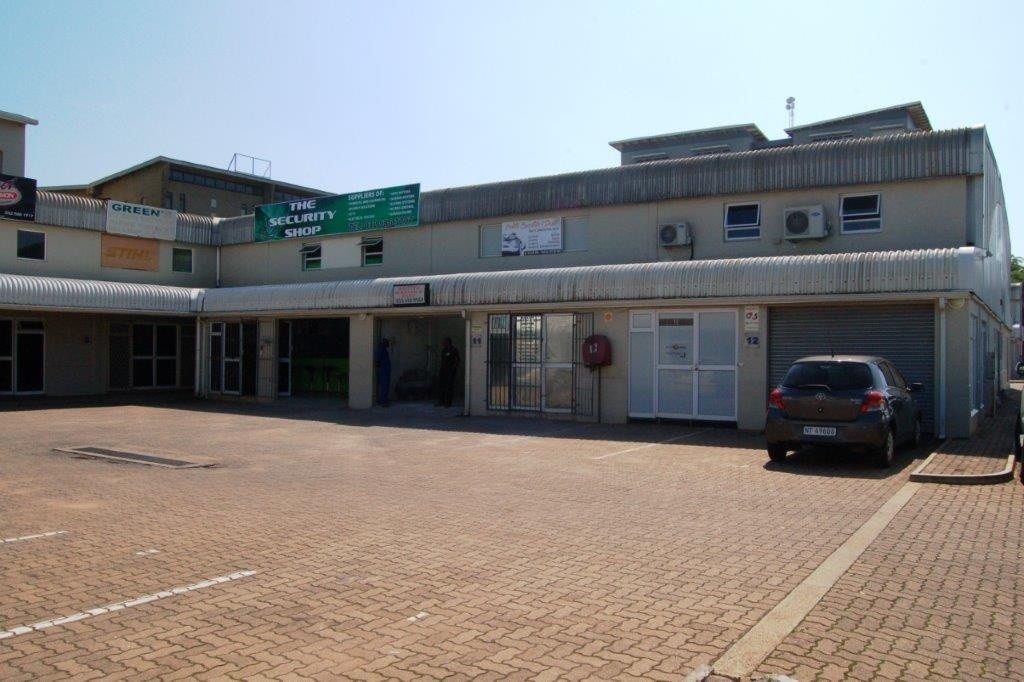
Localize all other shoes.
[375,398,390,406]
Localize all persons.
[377,339,392,404]
[434,337,459,406]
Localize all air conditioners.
[658,223,692,246]
[784,205,828,240]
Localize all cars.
[767,353,928,464]
